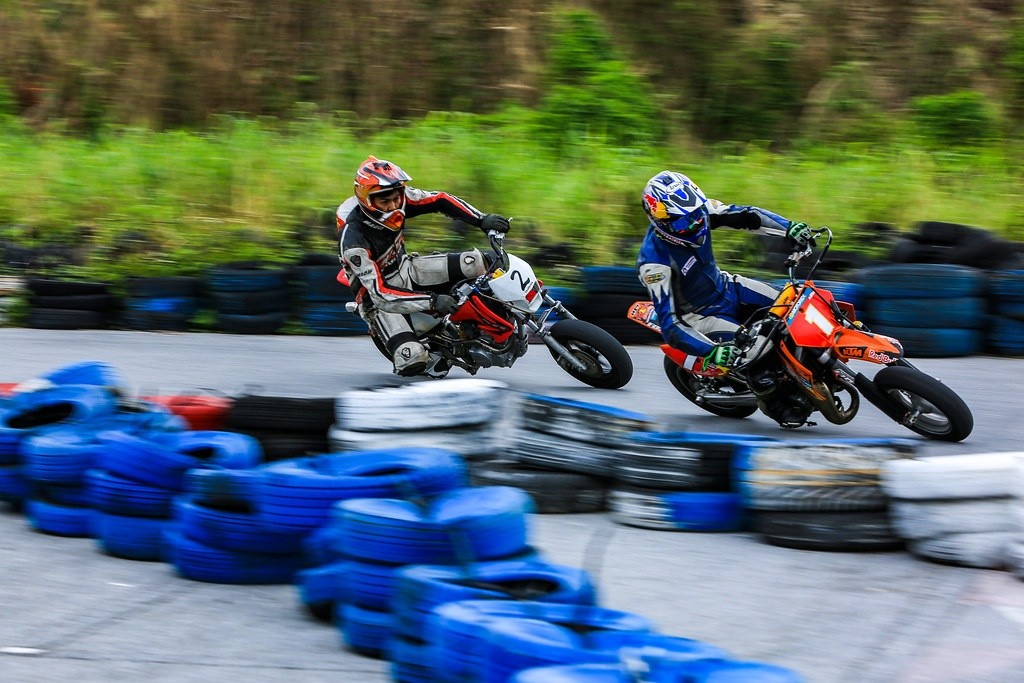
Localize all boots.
[747,376,810,425]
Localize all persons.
[638,170,817,423]
[338,157,511,379]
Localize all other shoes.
[426,353,450,380]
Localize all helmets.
[354,154,412,232]
[641,169,709,250]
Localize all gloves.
[712,344,747,368]
[479,213,511,235]
[433,295,460,317]
[785,221,817,248]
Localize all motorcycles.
[337,213,635,390]
[626,226,974,443]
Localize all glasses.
[667,206,704,231]
